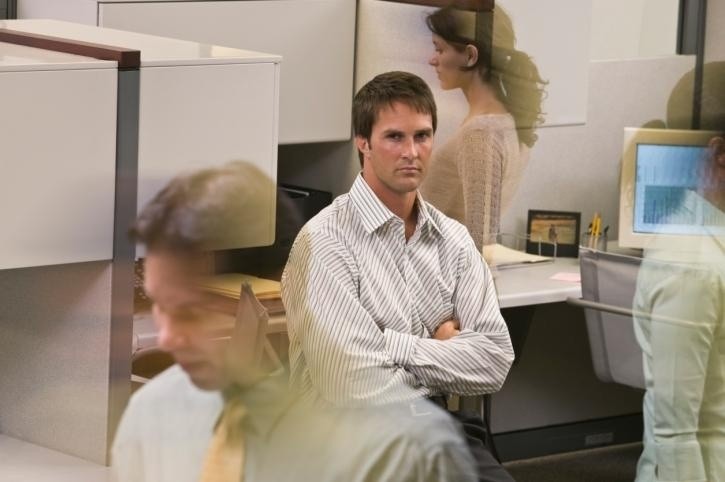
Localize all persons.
[105,158,476,482]
[617,60,724,482]
[281,70,526,482]
[416,3,545,282]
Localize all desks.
[132,240,639,464]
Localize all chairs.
[566,247,715,390]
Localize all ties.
[201,404,247,482]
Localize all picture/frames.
[526,209,581,258]
